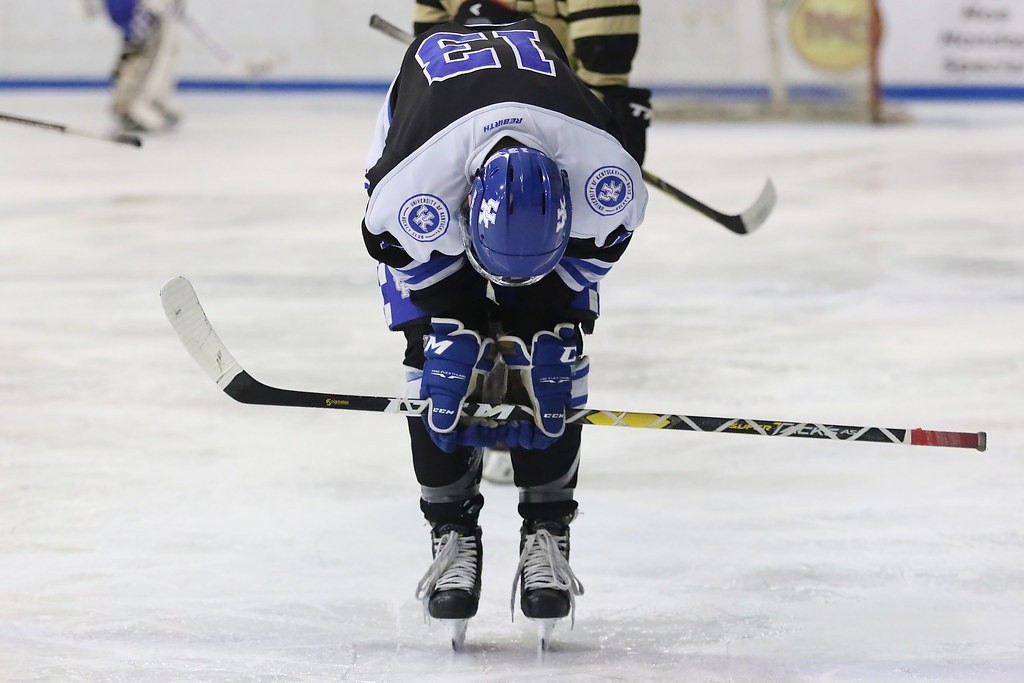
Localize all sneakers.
[511,500,584,655]
[415,494,484,650]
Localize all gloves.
[495,323,572,450]
[420,317,496,453]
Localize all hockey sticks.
[154,270,990,454]
[0,113,143,149]
[365,12,779,237]
[170,10,282,76]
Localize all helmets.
[457,146,572,285]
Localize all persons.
[103,0,187,131]
[361,0,653,655]
